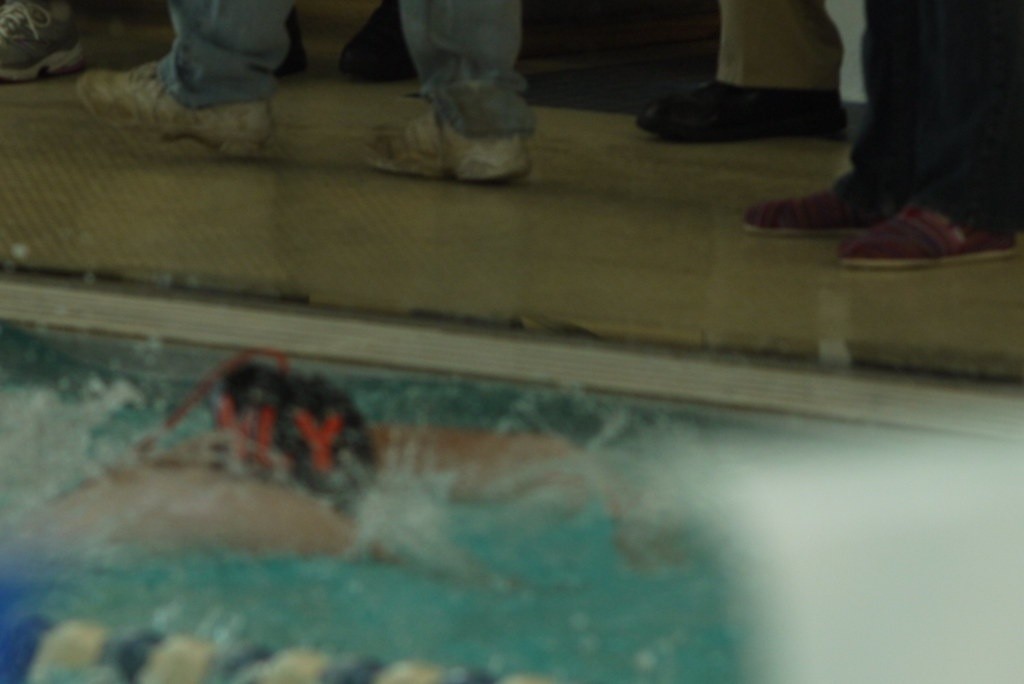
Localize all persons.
[744,0,1024,266]
[0,0,535,179]
[637,0,847,140]
[19,348,581,558]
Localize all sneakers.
[74,59,273,161]
[359,108,532,186]
[0,0,88,85]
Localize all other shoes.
[634,77,850,143]
[740,173,905,240]
[835,200,1020,271]
